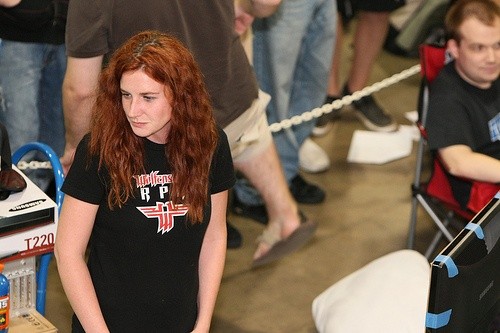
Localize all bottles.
[0,264,10,333]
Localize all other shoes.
[290,174,326,205]
[342,82,397,132]
[298,137,330,171]
[311,94,344,137]
[226,220,242,248]
[231,188,307,224]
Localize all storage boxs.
[0,164,61,333]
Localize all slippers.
[250,222,317,268]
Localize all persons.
[54,32,236,333]
[0,1,409,271]
[425,1,500,216]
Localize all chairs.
[406,44,495,262]
